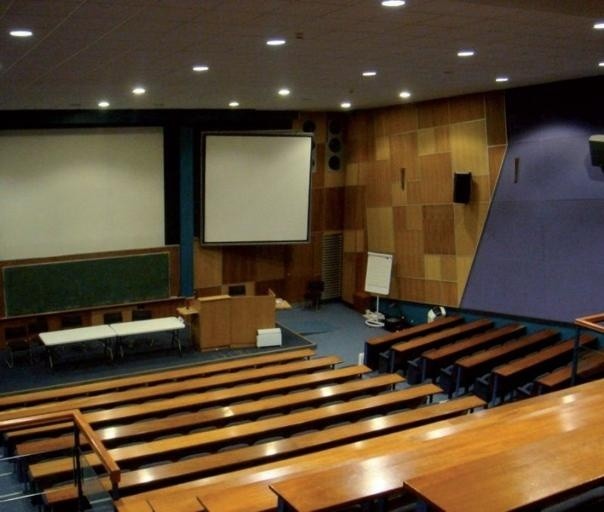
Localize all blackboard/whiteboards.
[3,250,171,317]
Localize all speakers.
[586,134,603,165]
[452,171,471,205]
